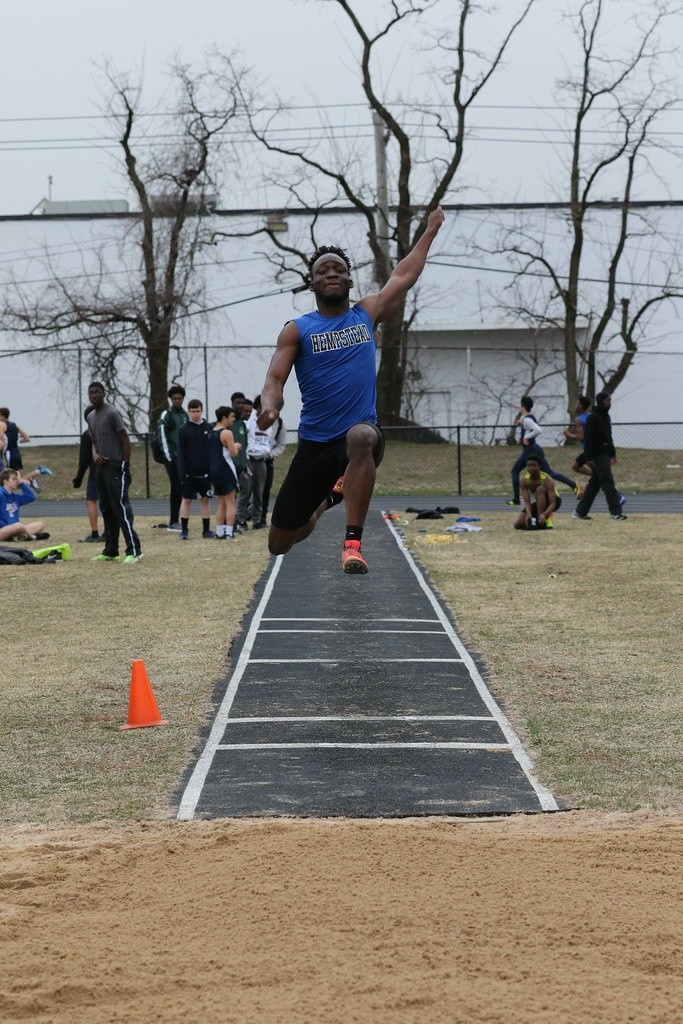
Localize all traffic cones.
[118,658,169,730]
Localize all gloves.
[72,476,82,488]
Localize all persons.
[572,392,627,521]
[72,406,106,543]
[514,454,561,530]
[0,408,52,493]
[86,381,144,565]
[506,396,583,505]
[563,397,626,508]
[157,387,286,541]
[0,469,50,542]
[256,206,444,575]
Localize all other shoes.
[13,534,30,541]
[166,515,268,541]
[78,534,101,543]
[32,532,50,540]
[617,495,627,506]
[101,534,106,542]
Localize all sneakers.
[37,465,54,476]
[610,513,628,522]
[333,477,345,495]
[91,553,121,562]
[506,499,521,506]
[545,519,553,528]
[30,477,42,493]
[123,553,143,565]
[572,509,592,521]
[342,540,368,574]
[573,481,582,499]
